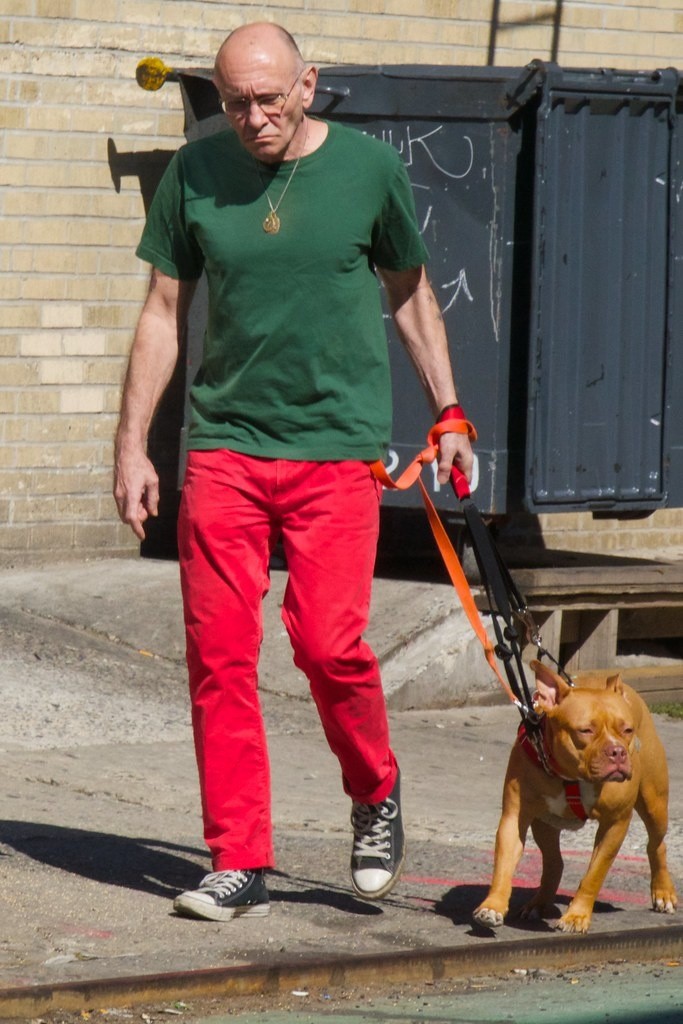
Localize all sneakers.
[173,866,270,922]
[349,767,406,900]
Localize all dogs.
[474,658,679,936]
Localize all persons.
[113,22,477,923]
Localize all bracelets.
[437,404,465,422]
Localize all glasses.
[218,66,306,118]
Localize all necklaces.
[251,118,308,234]
